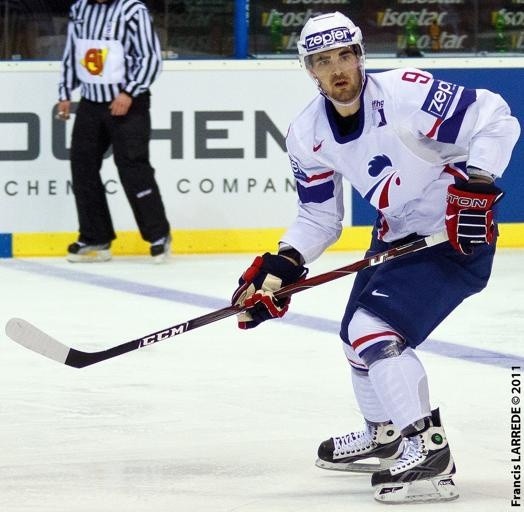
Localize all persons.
[232,10,522,503]
[57,0,174,263]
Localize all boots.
[67,240,110,255]
[317,406,456,488]
[150,233,172,256]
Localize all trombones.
[4,227,449,369]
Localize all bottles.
[496,10,507,53]
[430,19,441,50]
[272,10,282,53]
[406,15,419,57]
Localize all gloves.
[231,252,309,329]
[444,181,506,256]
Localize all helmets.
[296,11,366,71]
